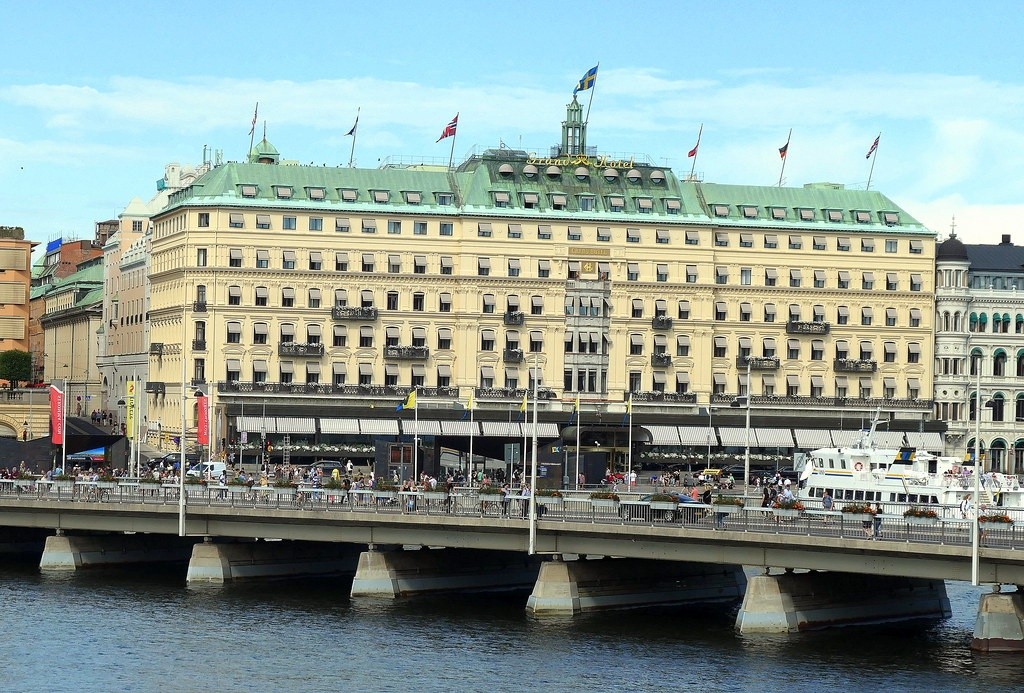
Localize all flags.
[866,137,879,159]
[573,65,597,96]
[436,116,458,144]
[396,390,630,426]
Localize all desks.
[0,478,15,495]
[36,481,54,502]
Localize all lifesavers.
[855,462,863,472]
[1007,477,1012,486]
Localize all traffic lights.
[260,441,264,452]
[222,438,225,447]
[266,440,270,446]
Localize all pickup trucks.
[147,452,204,470]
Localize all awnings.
[970,312,1024,404]
[229,165,923,390]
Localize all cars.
[684,468,722,486]
[721,465,746,478]
[618,492,707,523]
[749,465,799,486]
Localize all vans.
[185,461,226,480]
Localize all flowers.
[374,485,396,492]
[588,491,619,501]
[978,515,1011,523]
[840,506,876,514]
[772,496,803,510]
[714,498,744,506]
[426,485,448,492]
[16,471,33,479]
[479,486,506,495]
[653,494,678,502]
[535,490,562,497]
[55,473,297,482]
[903,509,936,518]
[324,477,341,489]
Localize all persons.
[862,501,883,541]
[47,460,174,485]
[754,474,791,491]
[979,504,988,547]
[959,494,972,530]
[624,470,637,485]
[394,465,531,519]
[76,402,82,417]
[605,466,610,479]
[660,469,735,489]
[681,484,712,513]
[761,486,795,514]
[23,430,28,441]
[0,461,31,489]
[215,460,374,502]
[822,492,836,525]
[579,472,584,488]
[713,494,727,531]
[90,409,117,427]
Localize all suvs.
[297,460,346,480]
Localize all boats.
[799,405,1024,532]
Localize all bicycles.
[437,502,464,515]
[87,487,109,503]
[167,487,177,501]
[291,491,313,510]
[473,502,499,515]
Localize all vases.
[17,479,34,486]
[374,491,398,498]
[478,493,504,502]
[56,483,298,488]
[906,516,937,525]
[980,522,1012,529]
[772,507,802,518]
[650,501,678,510]
[425,492,448,500]
[713,504,741,514]
[590,498,617,506]
[324,488,346,496]
[842,512,873,521]
[535,496,560,504]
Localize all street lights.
[115,368,135,478]
[178,359,205,537]
